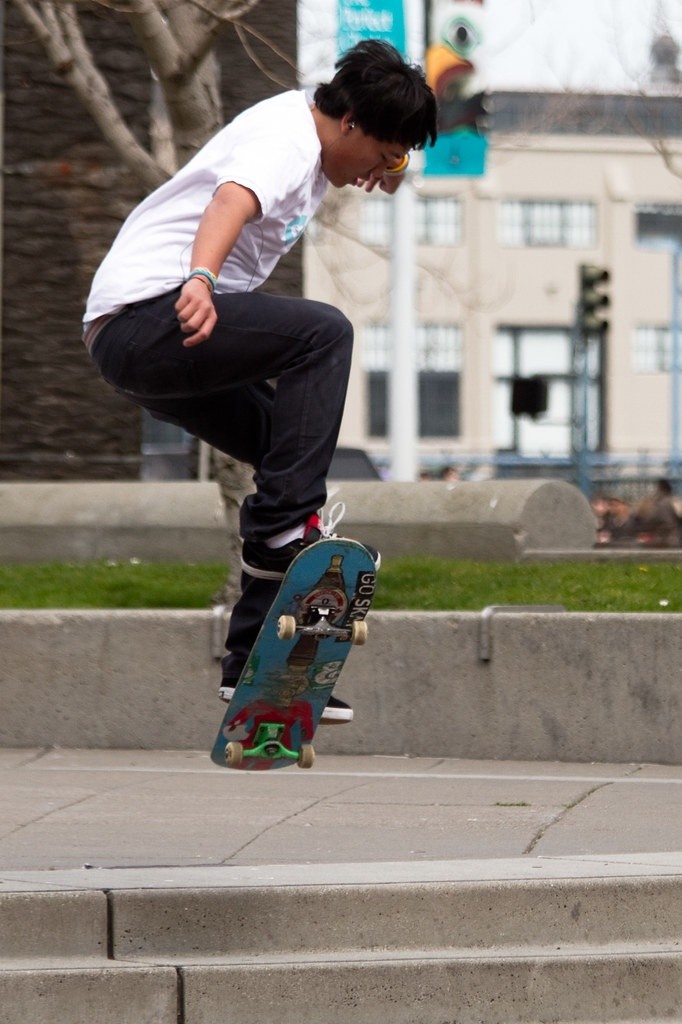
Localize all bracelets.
[384,149,410,177]
[188,267,218,300]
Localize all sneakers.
[217,677,353,726]
[241,510,381,583]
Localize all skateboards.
[211,537,378,774]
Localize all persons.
[79,36,495,732]
[374,453,682,557]
